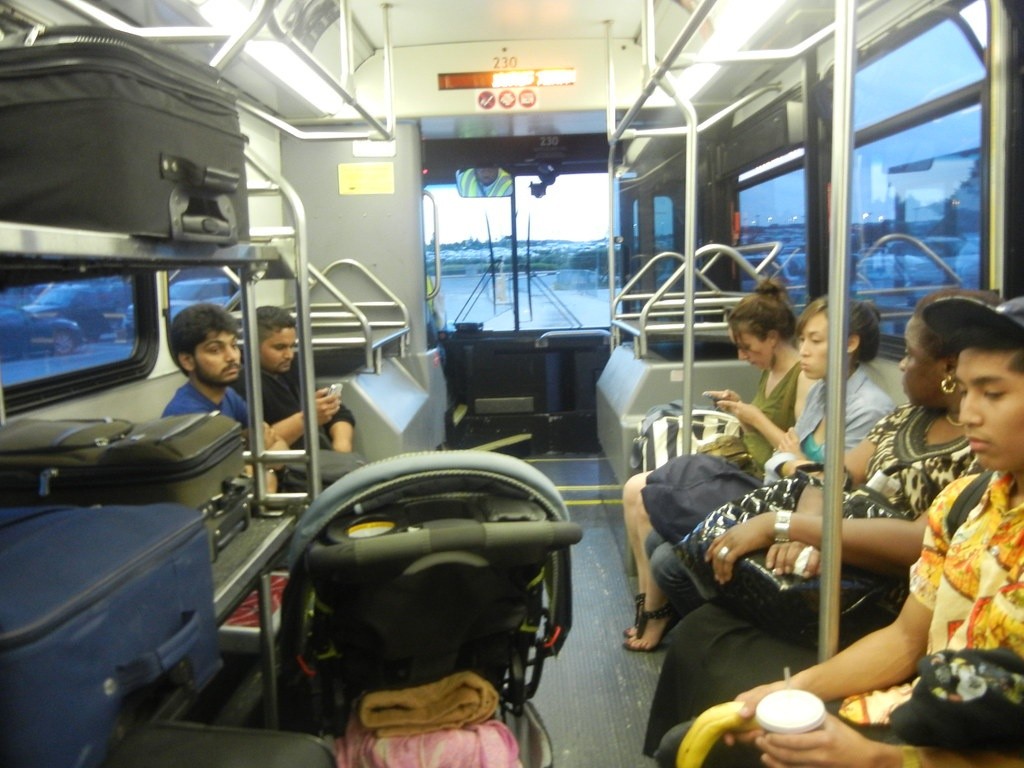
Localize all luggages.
[0,504,224,753]
[110,713,337,768]
[0,414,256,564]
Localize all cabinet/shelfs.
[2,149,320,729]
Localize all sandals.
[622,600,687,652]
[623,594,646,636]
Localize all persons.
[427,274,445,331]
[648,293,1024,767]
[157,303,367,492]
[643,293,894,618]
[620,274,815,653]
[637,285,1003,757]
[228,305,359,450]
[457,168,513,197]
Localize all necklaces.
[946,414,964,426]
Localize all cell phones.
[703,393,724,403]
[328,383,343,397]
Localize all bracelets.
[774,509,791,542]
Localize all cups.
[756,690,826,738]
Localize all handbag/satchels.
[674,462,938,649]
[641,453,766,546]
[640,410,741,475]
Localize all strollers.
[279,449,583,768]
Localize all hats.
[921,297,1024,339]
[888,649,1024,760]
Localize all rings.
[718,546,730,560]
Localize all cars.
[123,277,238,341]
[0,277,132,360]
[738,231,982,284]
